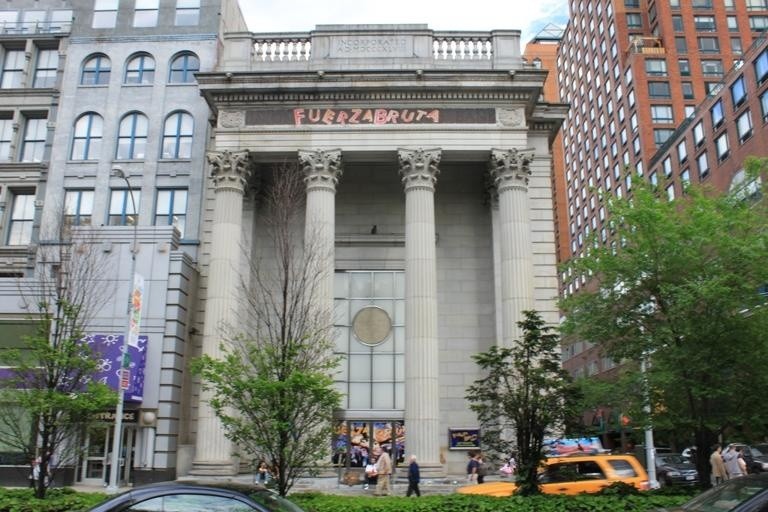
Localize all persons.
[467,449,518,483]
[372,445,393,496]
[406,454,421,497]
[364,456,378,491]
[708,443,750,487]
[254,461,269,485]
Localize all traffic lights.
[618,412,632,428]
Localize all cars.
[581,446,634,476]
[635,471,768,511]
[84,479,304,511]
[681,441,720,465]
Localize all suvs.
[721,440,768,474]
[453,452,650,497]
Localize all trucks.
[497,436,604,477]
[631,444,699,492]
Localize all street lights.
[611,264,660,490]
[104,166,138,492]
